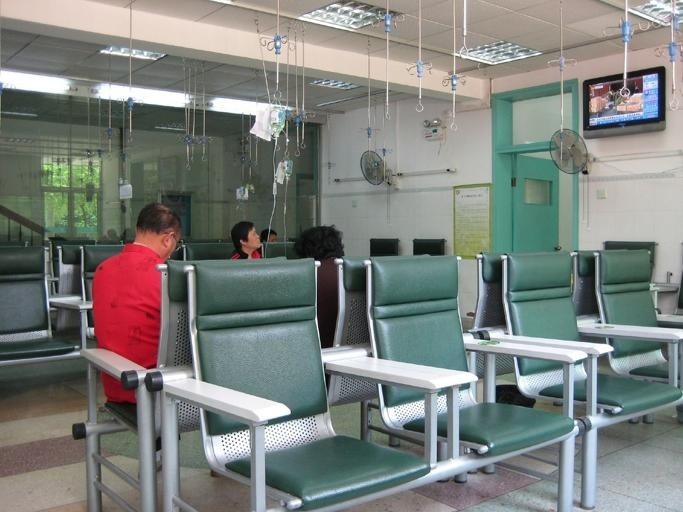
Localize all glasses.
[164,232,183,252]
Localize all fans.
[549,128,592,174]
[360,150,392,186]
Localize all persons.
[260,229,277,242]
[292,224,345,348]
[230,221,262,259]
[91,202,182,405]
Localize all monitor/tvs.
[582,65,667,139]
